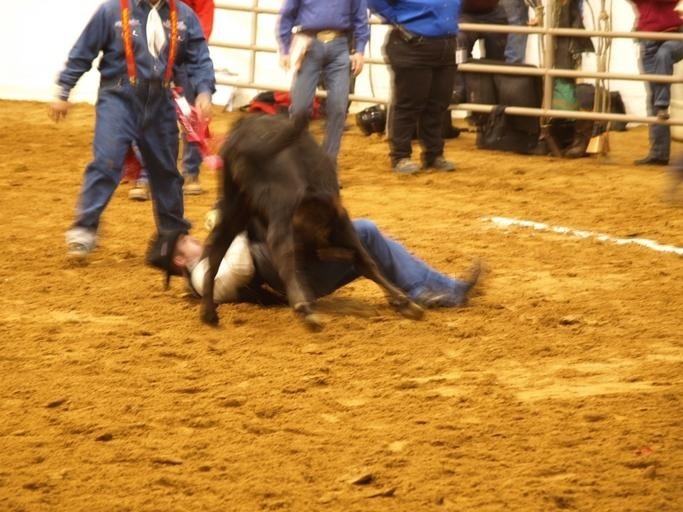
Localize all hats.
[148,227,187,290]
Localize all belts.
[316,29,344,42]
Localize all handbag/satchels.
[240,89,327,119]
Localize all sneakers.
[67,242,87,261]
[421,259,480,306]
[658,107,670,118]
[392,158,422,173]
[128,181,149,200]
[636,156,668,165]
[428,156,455,171]
[182,176,202,195]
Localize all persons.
[135,214,486,317]
[125,1,215,200]
[44,0,223,264]
[455,0,598,84]
[627,0,682,167]
[273,0,373,188]
[365,0,466,180]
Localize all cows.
[199,107,425,333]
